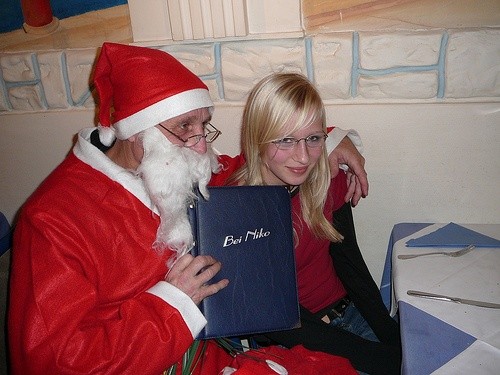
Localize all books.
[186,185,303,339]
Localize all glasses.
[159,123,222,146]
[271,132,328,150]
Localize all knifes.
[407,290,500,309]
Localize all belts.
[318,296,352,324]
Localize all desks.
[380,222,500,375]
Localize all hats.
[93,41,216,146]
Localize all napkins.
[406,222,500,247]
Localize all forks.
[398,244,475,260]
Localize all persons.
[8,42,359,375]
[219,73,402,375]
